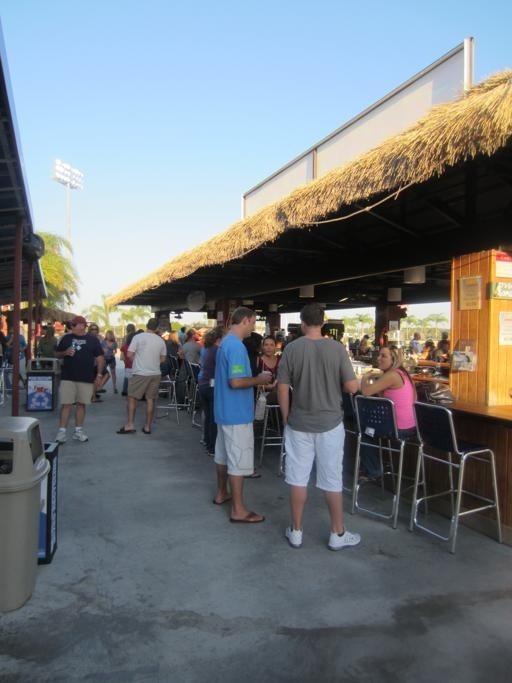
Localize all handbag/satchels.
[254,384,266,420]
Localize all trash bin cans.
[25,357,61,411]
[0,416,51,613]
[37,441,60,565]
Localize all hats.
[69,315,85,324]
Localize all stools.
[257,402,284,462]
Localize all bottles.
[254,394,267,421]
[69,341,76,357]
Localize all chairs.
[342,392,385,497]
[349,395,429,528]
[151,353,208,429]
[408,401,503,554]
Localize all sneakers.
[328,530,361,551]
[285,525,303,548]
[72,430,88,441]
[55,430,67,442]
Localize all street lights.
[53,158,84,316]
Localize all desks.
[409,372,448,397]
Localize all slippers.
[229,511,265,522]
[92,399,104,402]
[116,426,136,433]
[244,471,261,478]
[142,426,151,433]
[213,497,232,504]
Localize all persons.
[409,331,450,375]
[54,318,105,444]
[213,306,265,523]
[115,325,286,477]
[101,331,118,393]
[276,304,361,550]
[355,345,417,483]
[359,336,372,355]
[40,321,118,402]
[1,321,29,390]
